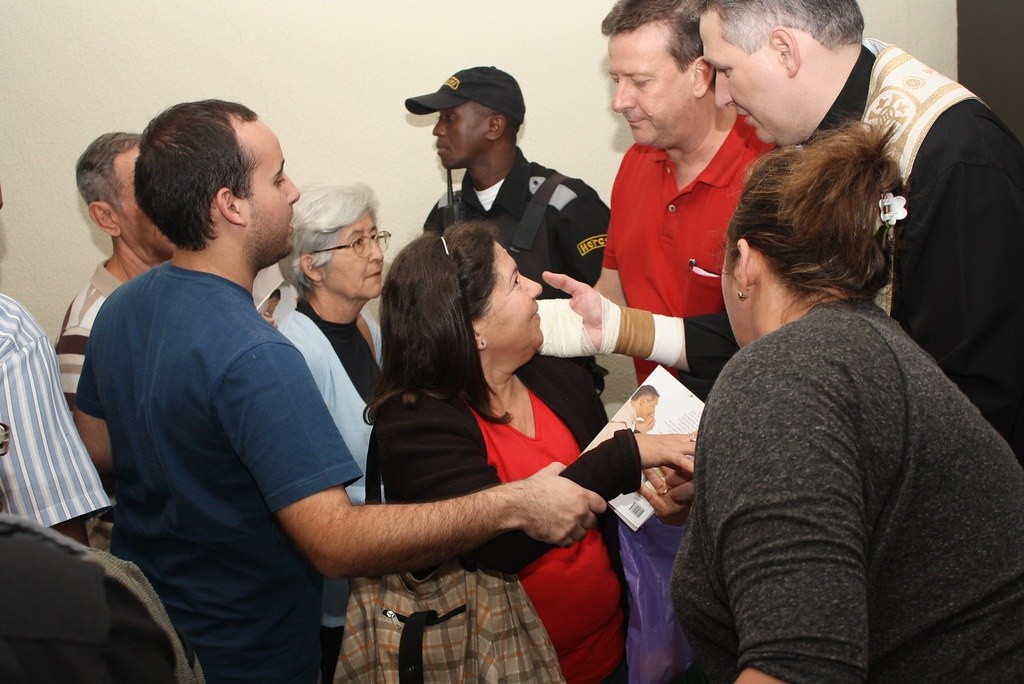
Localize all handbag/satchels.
[613,508,694,684]
[334,422,568,684]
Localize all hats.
[405,66,526,124]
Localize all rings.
[661,489,671,495]
[691,439,696,441]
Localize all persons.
[70,99,607,683]
[367,224,700,682]
[405,66,611,456]
[57,132,279,551]
[0,187,114,548]
[274,184,391,684]
[536,0,1024,468]
[667,124,1024,683]
[0,425,204,683]
[587,385,659,450]
[265,289,281,316]
[593,1,776,386]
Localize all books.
[576,364,705,533]
[257,281,294,332]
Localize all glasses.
[316,230,392,259]
